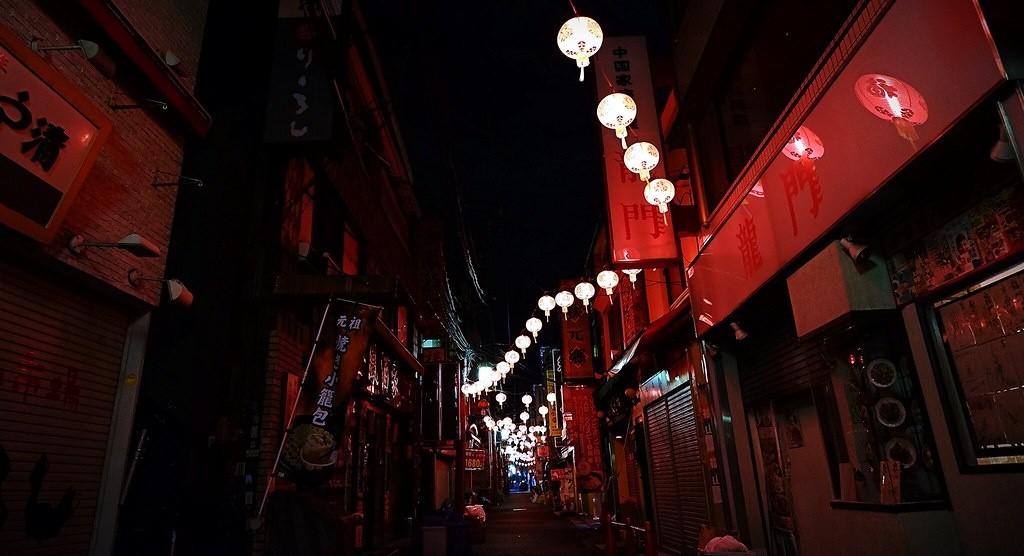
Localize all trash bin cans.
[422,526,447,556]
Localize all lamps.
[840,234,871,262]
[165,49,191,78]
[68,231,161,259]
[990,126,1012,162]
[128,268,192,306]
[730,321,748,341]
[30,38,99,59]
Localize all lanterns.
[483,393,549,467]
[594,94,676,226]
[781,125,826,161]
[461,269,643,403]
[557,17,604,84]
[855,73,930,141]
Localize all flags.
[276,298,381,489]
